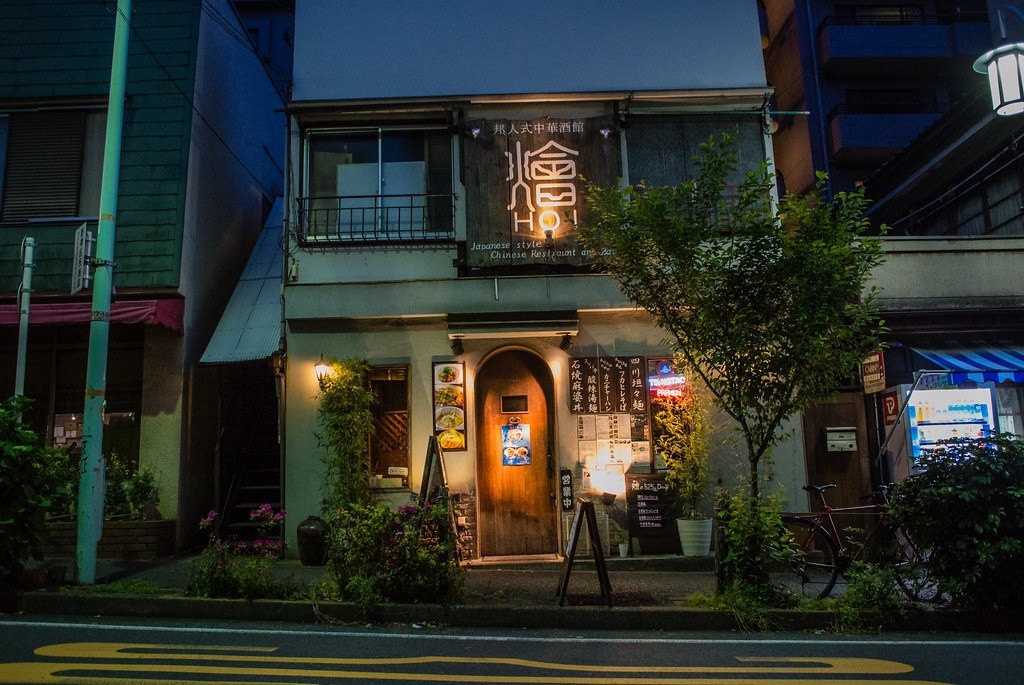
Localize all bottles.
[915,402,988,465]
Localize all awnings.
[874,339,1024,467]
[0,298,185,335]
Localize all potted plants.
[615,531,628,558]
[653,341,718,557]
[309,357,380,549]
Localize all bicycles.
[752,481,944,610]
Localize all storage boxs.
[54,422,83,446]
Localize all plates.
[503,429,529,458]
[434,362,464,449]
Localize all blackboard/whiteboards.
[410,436,465,568]
[555,497,612,608]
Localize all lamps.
[556,332,574,352]
[450,335,464,356]
[314,353,329,391]
